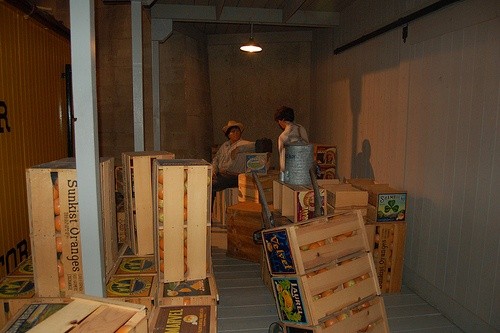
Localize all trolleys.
[252,166,323,333]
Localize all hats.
[222,120,245,134]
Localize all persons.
[211,121,256,226]
[275,106,309,174]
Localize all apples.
[53,184,65,292]
[157,170,211,275]
[299,230,382,333]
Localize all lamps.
[240,24,263,52]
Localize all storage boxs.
[214,143,409,333]
[0,149,220,333]
